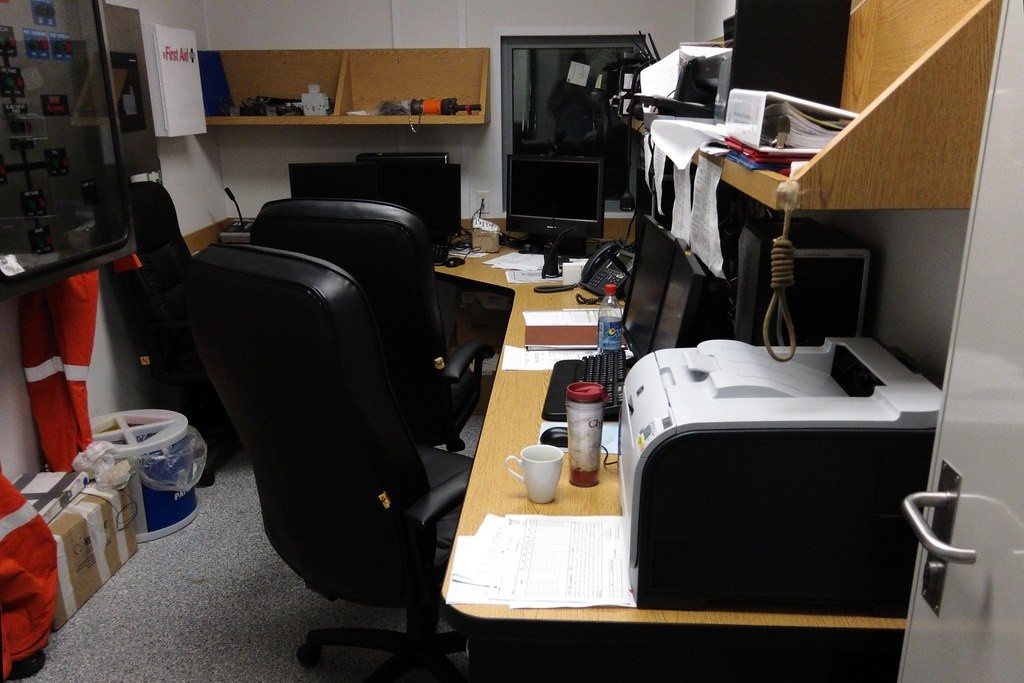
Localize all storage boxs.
[472,228,499,252]
[42,479,139,631]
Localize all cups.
[505,444,563,504]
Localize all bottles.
[566,382,608,487]
[597,284,622,355]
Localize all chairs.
[185,244,474,683]
[247,192,495,453]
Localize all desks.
[198,237,912,683]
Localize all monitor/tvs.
[288,162,462,236]
[506,154,606,258]
[620,214,707,368]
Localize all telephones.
[578,243,636,299]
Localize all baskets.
[471,228,499,253]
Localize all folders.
[724,89,858,153]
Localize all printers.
[616,337,943,617]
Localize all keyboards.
[431,245,449,265]
[575,349,626,415]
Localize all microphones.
[224,188,244,227]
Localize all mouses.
[445,257,465,267]
[540,427,568,447]
